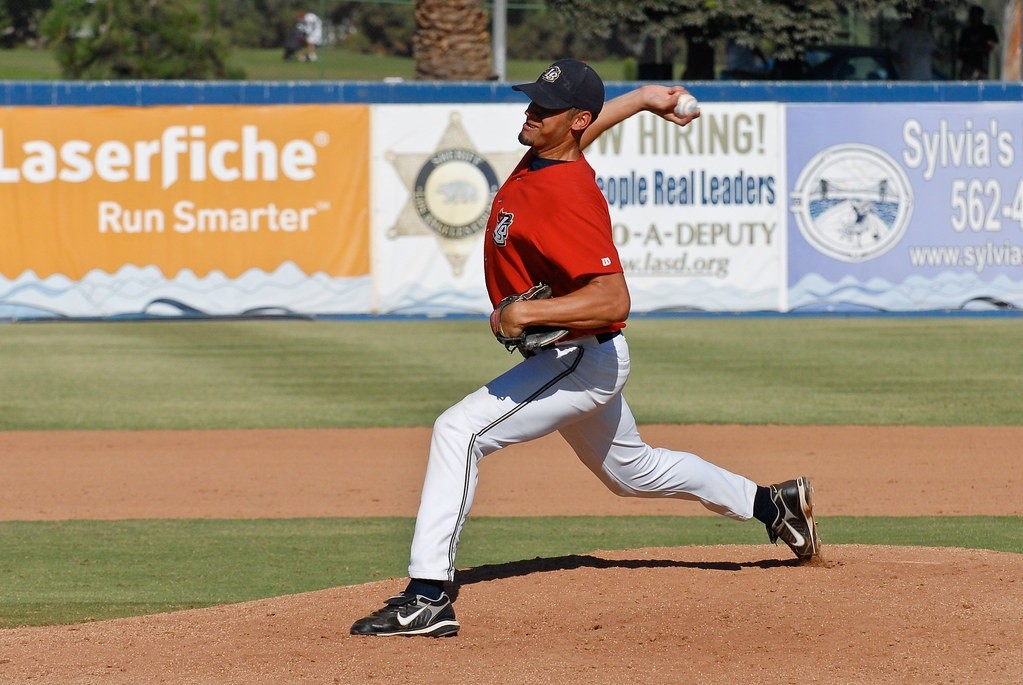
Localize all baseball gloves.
[489,282,569,352]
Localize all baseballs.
[673,93,699,119]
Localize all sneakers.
[350,591,460,638]
[766,476,821,563]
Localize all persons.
[349,58,820,638]
[297,11,322,62]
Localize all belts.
[524,330,621,358]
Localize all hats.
[512,59,605,113]
